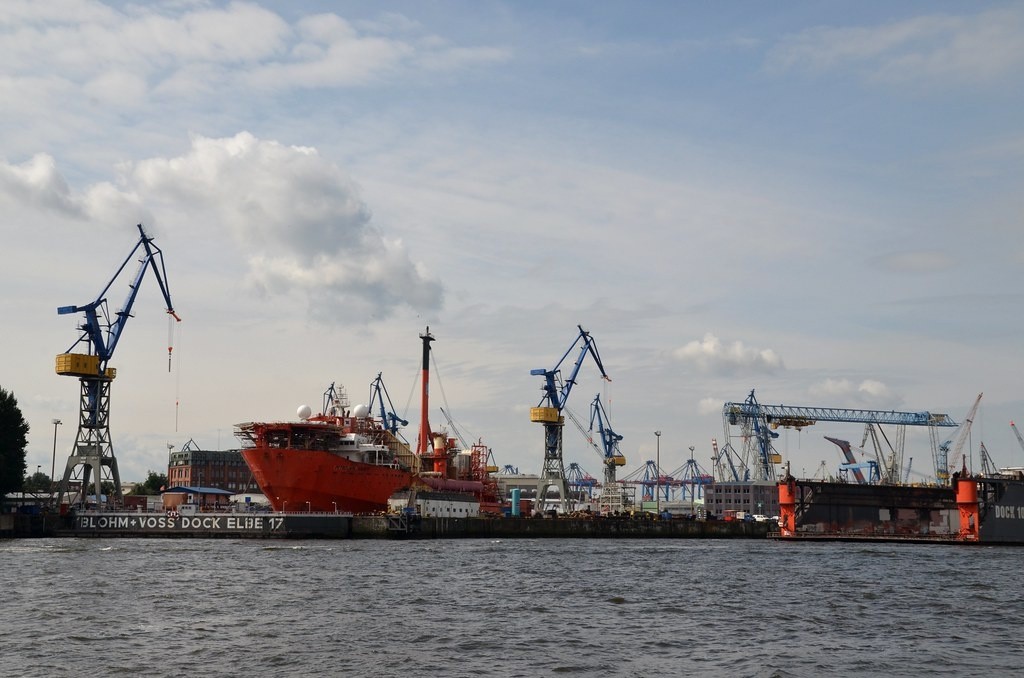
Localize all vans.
[752,514,769,522]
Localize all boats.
[231,384,421,513]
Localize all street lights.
[51,418,63,490]
[653,430,662,515]
[688,445,695,479]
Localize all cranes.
[528,323,630,466]
[47,219,183,378]
[708,391,1024,486]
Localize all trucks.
[736,511,756,523]
